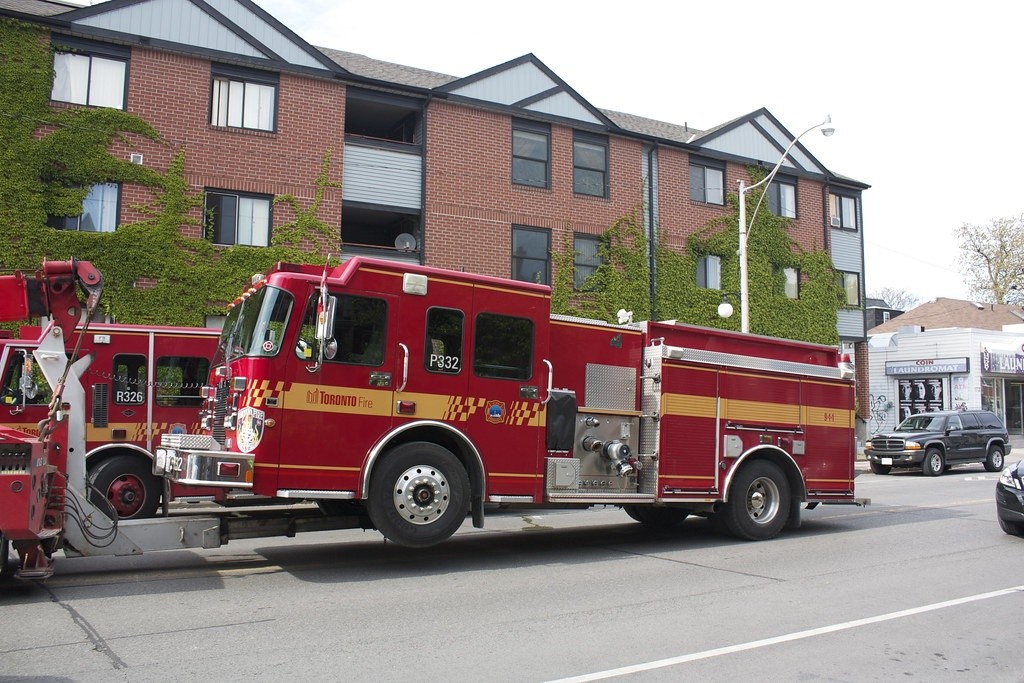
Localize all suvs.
[864,410,1011,478]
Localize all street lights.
[740,114,835,334]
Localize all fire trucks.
[0,321,222,520]
[0,256,377,581]
[152,252,871,542]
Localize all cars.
[995,459,1024,535]
